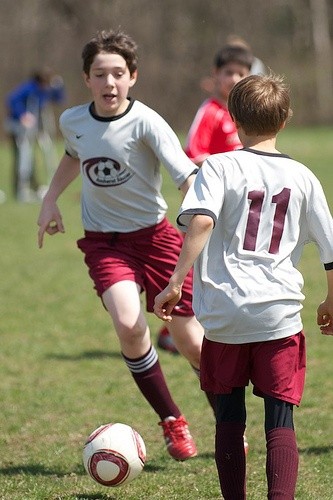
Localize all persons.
[158,45,264,353]
[154,74,333,500]
[5,64,67,203]
[37,30,248,461]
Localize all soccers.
[82,423,145,486]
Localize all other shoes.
[158,328,180,355]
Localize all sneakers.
[243,437,248,454]
[159,416,196,460]
